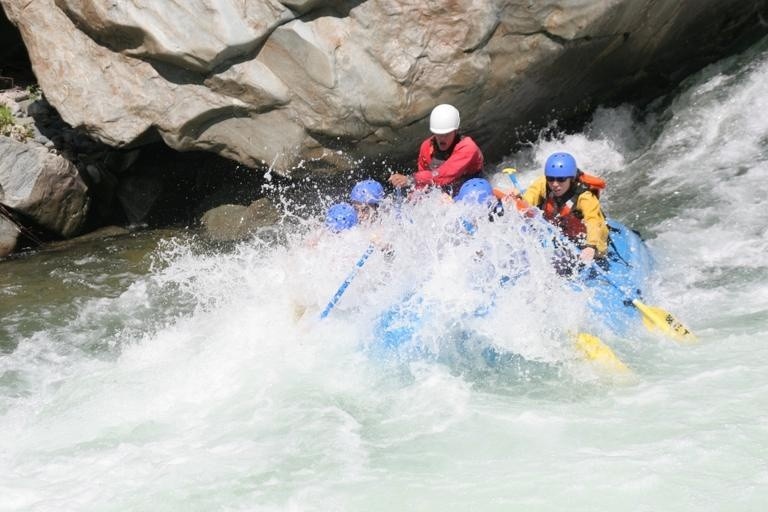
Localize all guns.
[505,169,631,384]
[530,202,698,351]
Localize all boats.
[367,216,661,400]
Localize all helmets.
[325,104,577,232]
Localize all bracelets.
[406,172,417,187]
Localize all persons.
[349,178,410,261]
[387,99,489,195]
[449,177,537,236]
[319,201,355,242]
[519,151,609,264]
